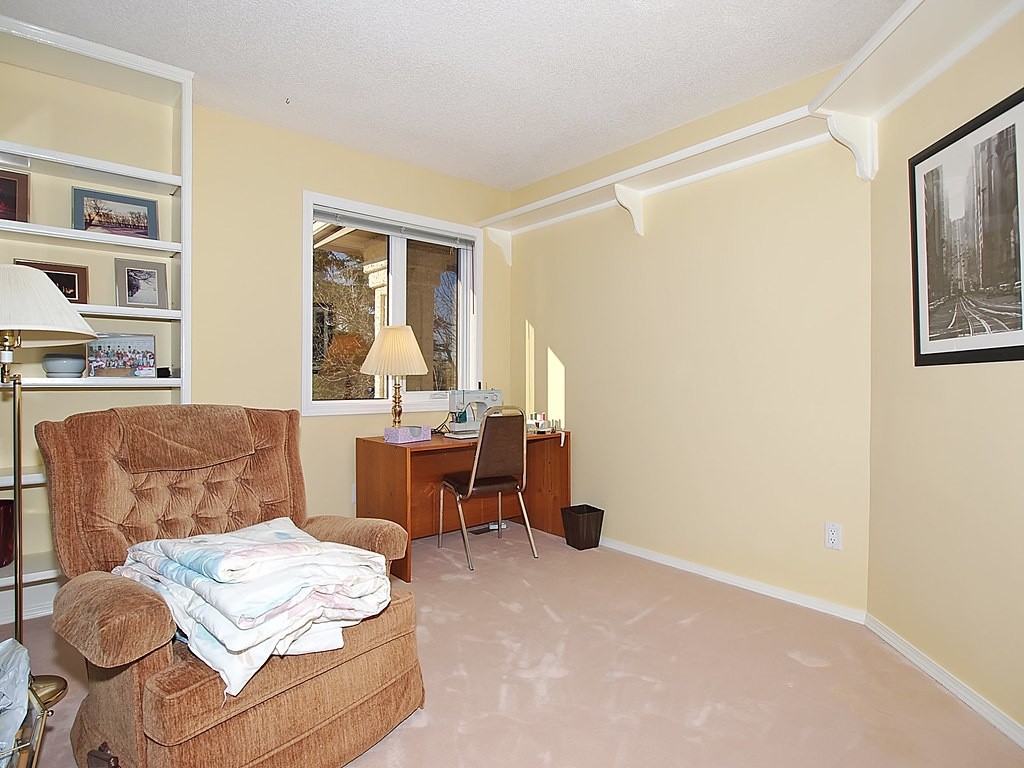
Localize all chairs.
[438,404,539,570]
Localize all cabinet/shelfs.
[0,14,194,625]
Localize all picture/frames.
[13,258,87,304]
[70,185,159,240]
[85,332,156,379]
[114,258,167,310]
[907,85,1024,366]
[0,166,30,223]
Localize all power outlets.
[824,521,841,551]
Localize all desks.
[355,429,570,582]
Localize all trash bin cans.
[561,505,604,550]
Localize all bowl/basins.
[42,354,86,377]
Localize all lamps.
[0,264,97,710]
[359,326,428,427]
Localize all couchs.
[34,404,425,767]
